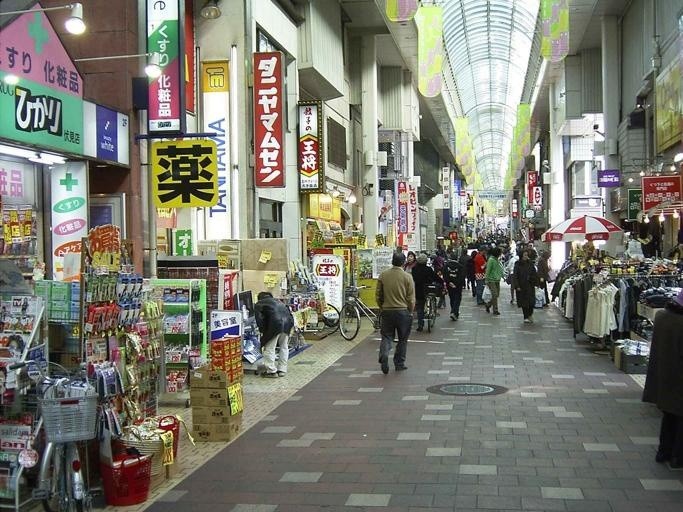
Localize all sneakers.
[492,311,500,315]
[449,312,458,321]
[523,316,533,324]
[484,304,490,313]
[477,301,486,305]
[415,325,423,332]
[278,370,286,377]
[259,371,278,378]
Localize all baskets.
[98,443,152,506]
[36,390,101,445]
[133,415,179,459]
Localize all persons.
[376,224,551,333]
[253,291,294,378]
[374,253,415,373]
[640,290,683,467]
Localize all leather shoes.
[394,364,408,371]
[380,355,389,374]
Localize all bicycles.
[422,282,443,333]
[8,357,119,511]
[339,284,412,344]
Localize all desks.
[257,307,312,359]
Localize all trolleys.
[182,354,213,408]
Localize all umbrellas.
[541,214,626,244]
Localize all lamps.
[324,176,356,203]
[200,0,221,19]
[644,214,650,223]
[658,213,665,222]
[74,52,163,79]
[0,3,86,35]
[673,210,679,218]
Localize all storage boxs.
[190,365,241,442]
